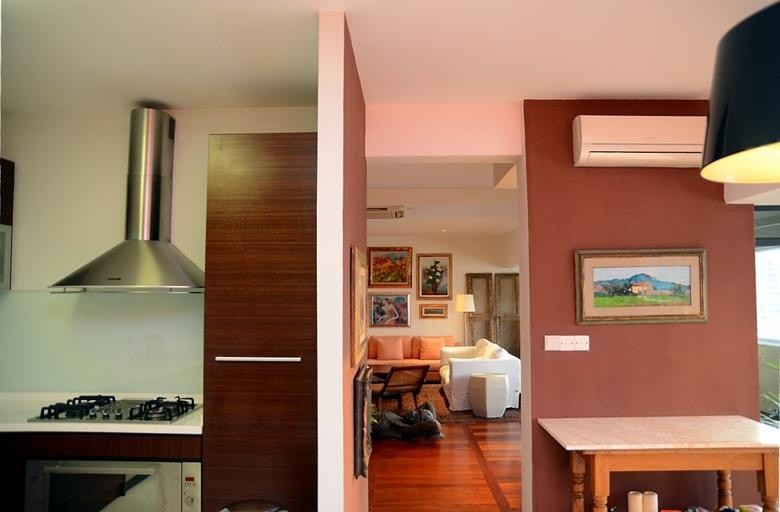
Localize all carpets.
[371,384,521,424]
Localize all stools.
[468,373,509,418]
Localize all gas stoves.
[26,393,199,422]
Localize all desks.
[538,414,780,512]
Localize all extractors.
[50,106,205,293]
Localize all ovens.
[25,460,201,512]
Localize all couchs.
[439,339,521,411]
[369,335,464,384]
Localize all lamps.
[454,293,475,346]
[700,0,780,185]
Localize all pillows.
[420,337,444,359]
[377,337,403,359]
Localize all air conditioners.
[367,205,406,221]
[572,115,708,168]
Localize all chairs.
[373,365,430,412]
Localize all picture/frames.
[416,253,453,300]
[368,247,413,288]
[369,292,411,327]
[419,304,448,319]
[352,243,369,368]
[575,247,708,325]
[353,363,374,480]
